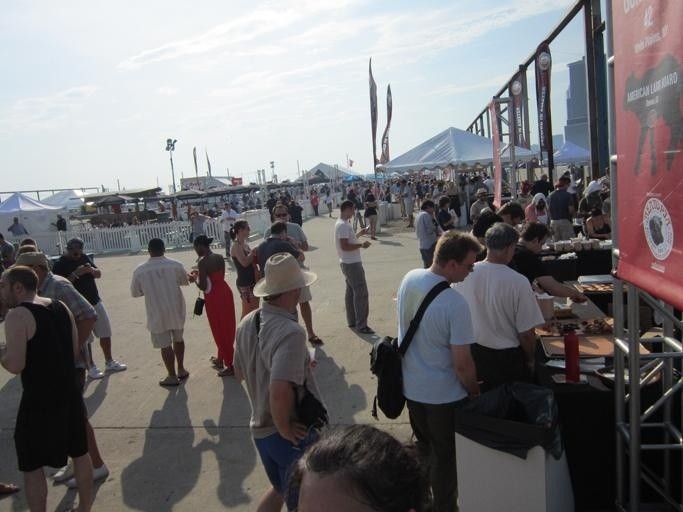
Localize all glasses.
[462,263,474,270]
[275,213,286,218]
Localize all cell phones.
[85,262,91,268]
[365,224,370,232]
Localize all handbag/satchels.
[368,335,405,421]
[295,384,328,429]
[193,297,204,315]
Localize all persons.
[130,192,323,388]
[96,198,261,228]
[282,416,439,512]
[0,233,128,511]
[508,222,588,303]
[311,168,611,269]
[334,200,376,335]
[397,224,545,512]
[232,252,318,511]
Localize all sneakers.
[159,375,179,386]
[218,367,233,376]
[104,360,126,370]
[87,367,104,379]
[53,457,74,481]
[177,367,188,378]
[211,356,223,369]
[66,463,108,488]
[0,482,17,494]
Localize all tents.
[1,189,89,236]
[313,170,332,182]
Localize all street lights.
[166,138,178,193]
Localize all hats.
[252,252,317,297]
[7,252,47,269]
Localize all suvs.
[68,208,81,220]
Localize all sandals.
[308,334,321,344]
[357,325,374,334]
